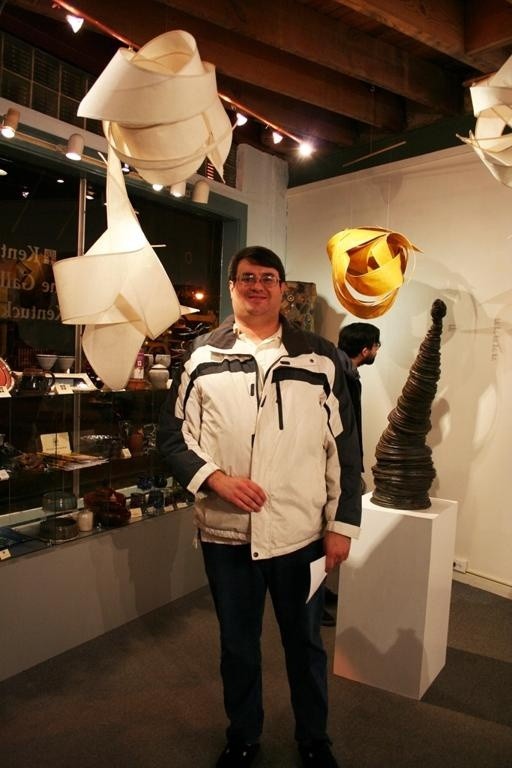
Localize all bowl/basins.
[35,354,57,371]
[56,356,76,372]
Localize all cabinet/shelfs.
[330,488,456,702]
[1,151,224,686]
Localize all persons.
[307,321,383,628]
[155,244,364,767]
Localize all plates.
[0,357,15,394]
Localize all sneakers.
[317,586,338,626]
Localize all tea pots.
[11,367,55,397]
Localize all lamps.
[1,108,212,205]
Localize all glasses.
[231,271,282,289]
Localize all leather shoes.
[293,730,342,766]
[213,740,266,768]
